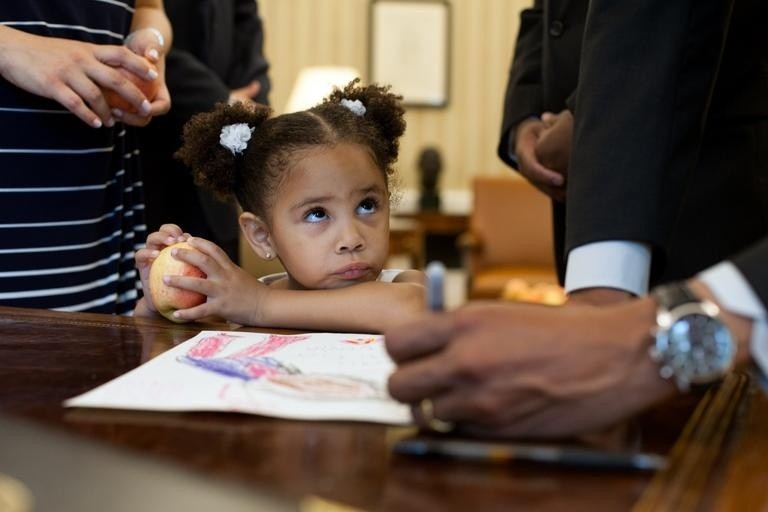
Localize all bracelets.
[123,26,166,48]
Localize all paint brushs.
[423,259,448,312]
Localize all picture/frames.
[366,0,451,108]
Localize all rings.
[421,399,455,434]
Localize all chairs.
[456,174,568,304]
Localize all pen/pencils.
[393,436,665,473]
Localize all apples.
[147,243,206,324]
[101,58,159,113]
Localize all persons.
[145,0,272,267]
[383,0,768,443]
[495,1,588,288]
[132,76,429,333]
[0,0,178,316]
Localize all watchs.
[650,284,737,394]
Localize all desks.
[0,302,767,512]
